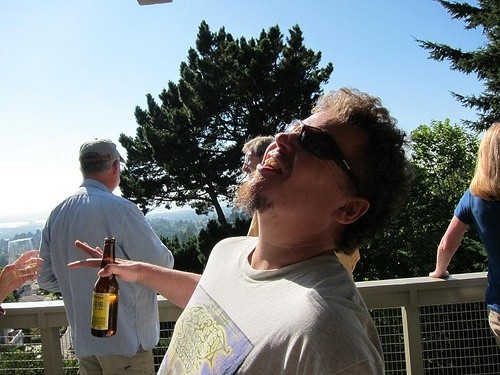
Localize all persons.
[0,250,44,317]
[68,87,413,375]
[429,122,500,349]
[37,137,175,375]
[242,136,361,282]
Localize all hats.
[79,138,126,163]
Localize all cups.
[7,237,39,286]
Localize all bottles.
[90,237,120,338]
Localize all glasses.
[284,117,363,197]
